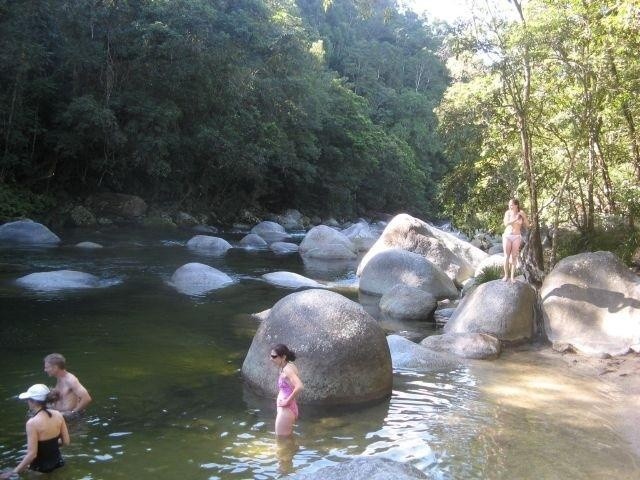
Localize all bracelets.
[71,409,76,414]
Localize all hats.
[19,384,50,402]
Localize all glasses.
[271,355,278,359]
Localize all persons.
[0,384,70,480]
[275,435,299,474]
[501,199,529,283]
[45,353,92,431]
[269,343,304,435]
